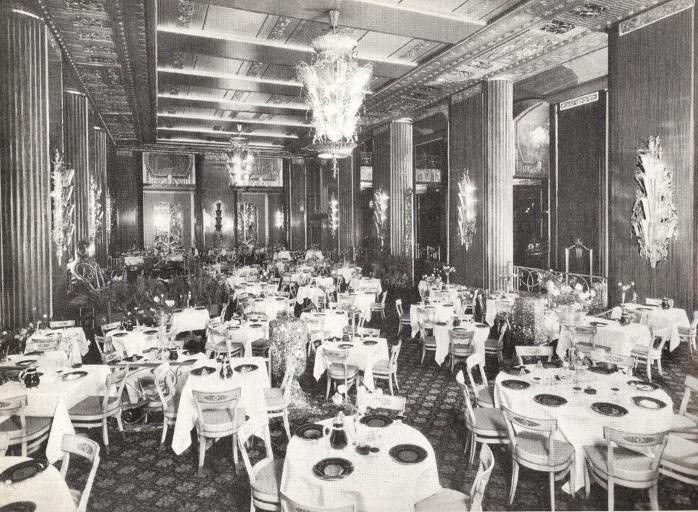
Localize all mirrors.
[512,98,551,273]
[289,160,307,251]
[349,142,375,248]
[414,116,449,266]
[238,193,269,247]
[142,187,192,249]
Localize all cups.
[355,427,383,457]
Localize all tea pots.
[619,310,633,327]
[122,320,133,331]
[343,331,353,342]
[219,356,234,380]
[453,316,461,326]
[323,419,349,450]
[661,296,672,309]
[18,364,41,389]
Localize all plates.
[121,347,200,366]
[250,293,285,302]
[143,330,158,335]
[219,315,268,335]
[296,423,329,439]
[0,458,50,483]
[388,443,426,463]
[0,500,37,512]
[114,332,128,338]
[361,414,393,427]
[15,360,36,367]
[315,314,326,317]
[436,322,447,326]
[313,457,355,479]
[234,363,260,372]
[363,341,378,345]
[327,337,354,349]
[500,361,667,416]
[190,366,215,375]
[61,370,87,381]
[475,324,487,328]
[636,303,657,311]
[590,321,607,327]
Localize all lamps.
[221,123,258,192]
[296,11,371,178]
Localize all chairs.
[236,392,496,509]
[395,274,697,373]
[457,346,698,509]
[101,249,403,470]
[3,300,129,510]
